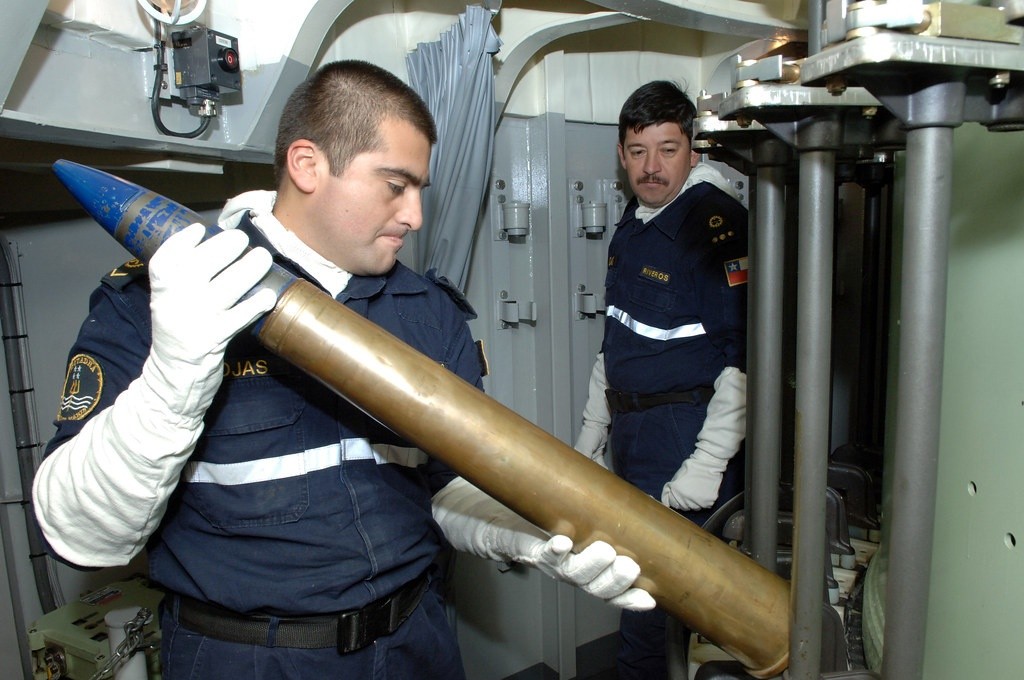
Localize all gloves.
[572,351,610,471]
[431,474,657,614]
[31,221,276,570]
[659,365,748,510]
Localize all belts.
[158,563,444,653]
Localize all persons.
[574,81,748,680]
[31,60,656,680]
